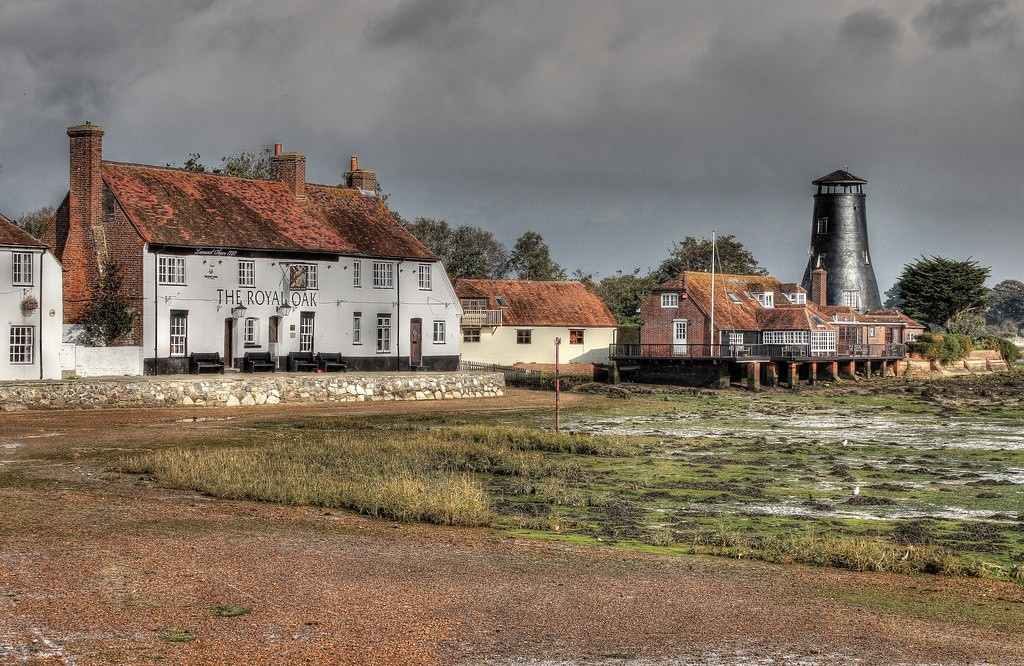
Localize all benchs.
[732,347,750,355]
[782,346,806,356]
[849,345,868,352]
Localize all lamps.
[276,299,292,316]
[230,300,247,317]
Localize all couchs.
[317,352,348,373]
[245,351,276,374]
[288,351,319,373]
[190,352,225,375]
